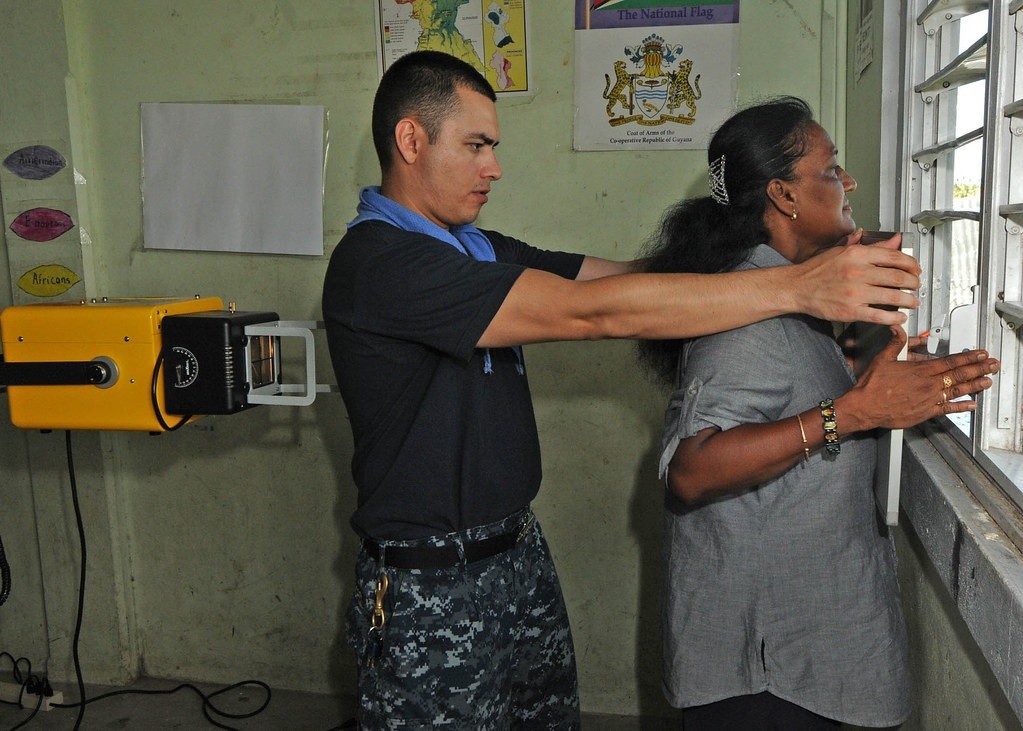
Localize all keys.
[365,640,379,669]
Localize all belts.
[363,514,536,569]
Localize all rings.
[942,389,948,402]
[942,372,952,389]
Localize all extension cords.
[0,681,63,712]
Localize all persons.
[635,94,1004,731]
[322,49,924,731]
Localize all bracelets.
[817,397,843,456]
[795,412,812,462]
